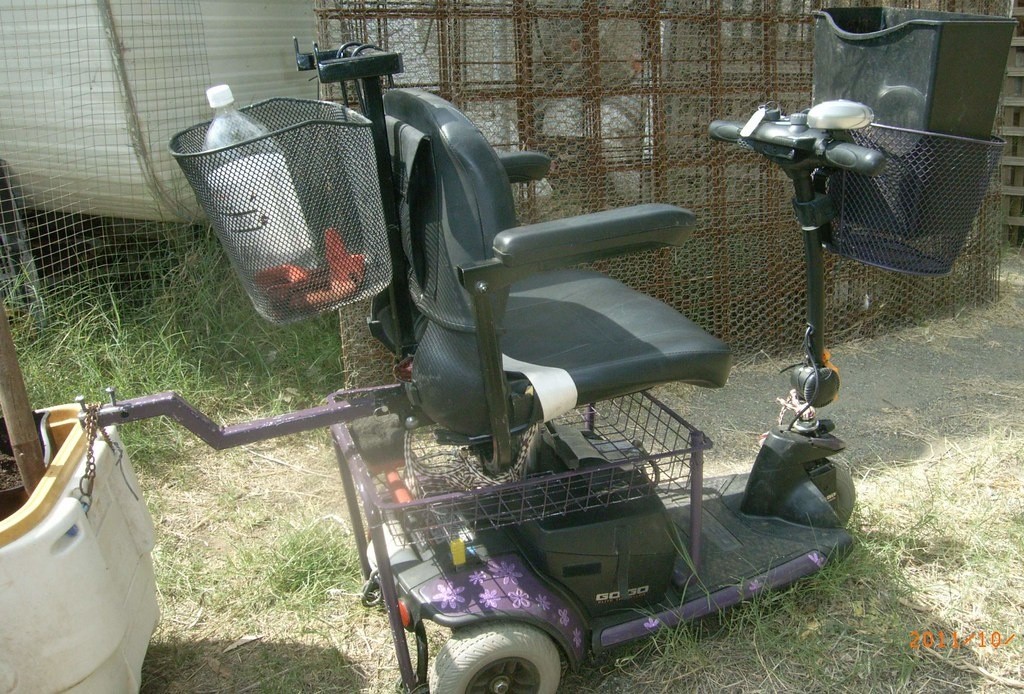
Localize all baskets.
[170,94,391,327]
[827,120,1009,274]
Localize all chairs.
[383,86,732,479]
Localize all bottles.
[198,84,318,281]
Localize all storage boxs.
[0,399,161,694]
[811,6,1019,238]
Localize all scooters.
[0,5,1023,694]
